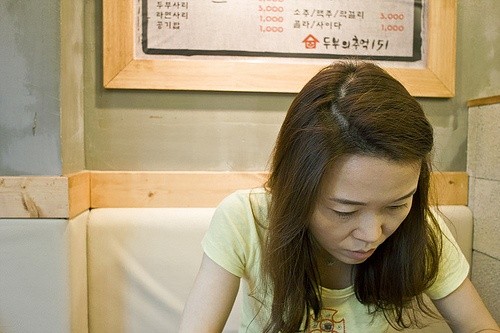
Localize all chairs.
[66,205,473,332]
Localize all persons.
[179,59,499,333]
